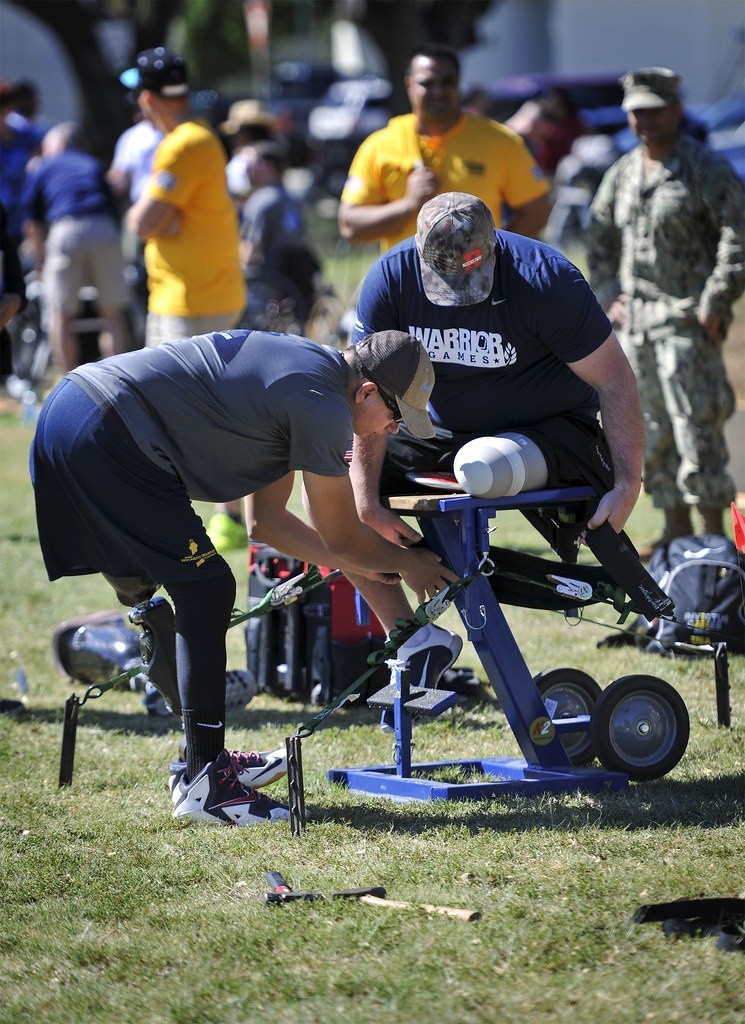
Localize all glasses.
[361,367,404,423]
[127,81,159,105]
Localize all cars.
[211,58,745,196]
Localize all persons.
[28,328,462,824]
[572,66,744,563]
[120,46,249,556]
[1,47,744,563]
[299,190,645,734]
[337,46,554,258]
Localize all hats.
[220,98,276,134]
[120,47,189,98]
[355,330,436,439]
[414,192,496,306]
[621,66,680,111]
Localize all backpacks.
[594,534,745,658]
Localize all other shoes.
[635,507,695,560]
[699,509,727,539]
[143,668,260,719]
[205,513,251,554]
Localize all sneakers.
[380,621,463,735]
[170,751,311,826]
[167,747,289,803]
